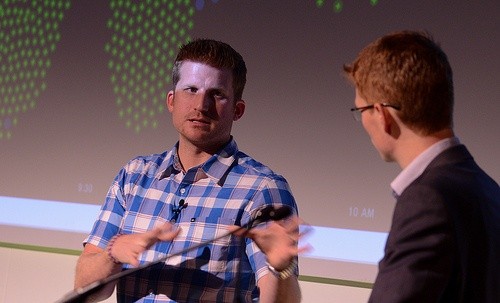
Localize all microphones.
[179,199,184,206]
[62,206,293,303]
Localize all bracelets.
[108,233,125,264]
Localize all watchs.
[268,259,298,281]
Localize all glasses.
[349,103,400,124]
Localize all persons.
[75,38,301,303]
[340,30,500,303]
